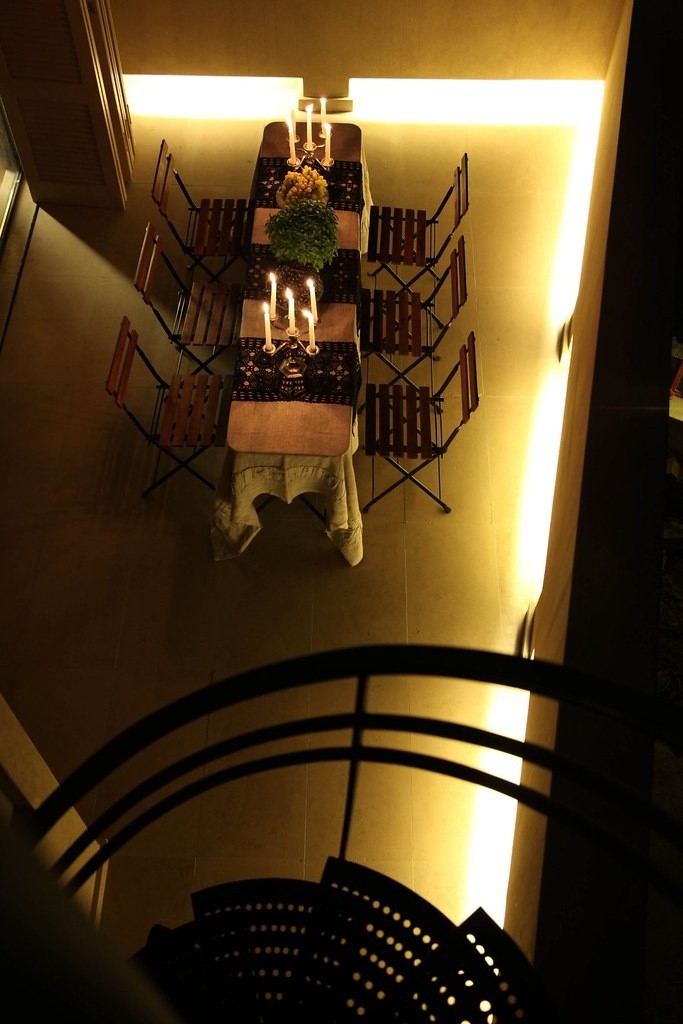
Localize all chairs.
[134,221,243,402]
[358,330,482,515]
[104,316,233,499]
[150,138,250,298]
[359,235,469,413]
[364,152,471,326]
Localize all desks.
[210,123,365,567]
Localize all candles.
[305,279,319,322]
[287,122,296,163]
[261,302,273,351]
[319,97,327,136]
[301,308,315,350]
[269,272,278,317]
[323,122,333,160]
[285,287,296,334]
[306,103,313,147]
[287,102,297,140]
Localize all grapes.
[282,166,327,210]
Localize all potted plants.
[264,198,339,333]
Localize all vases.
[276,187,329,209]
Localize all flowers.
[278,165,328,204]
